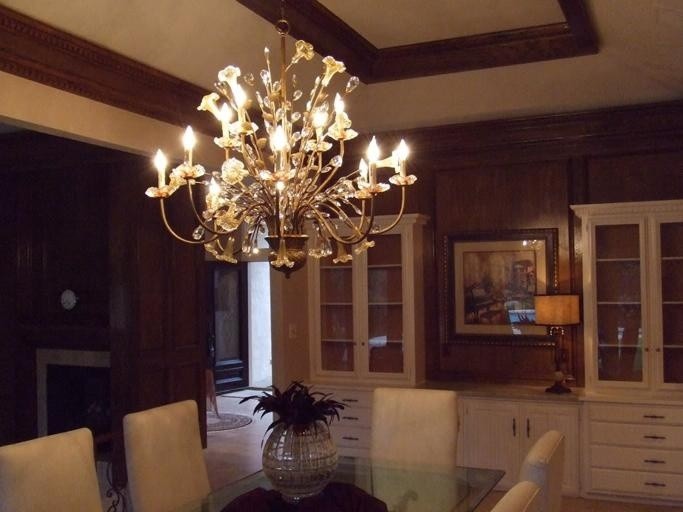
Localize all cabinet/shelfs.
[462,395,581,499]
[570,198,683,506]
[304,212,433,474]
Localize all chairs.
[369,389,462,466]
[515,428,564,508]
[1,423,107,511]
[118,397,214,509]
[489,478,539,511]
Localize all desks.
[155,445,505,508]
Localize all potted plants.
[238,378,352,499]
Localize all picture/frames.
[438,226,559,348]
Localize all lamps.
[145,5,417,279]
[533,292,579,393]
